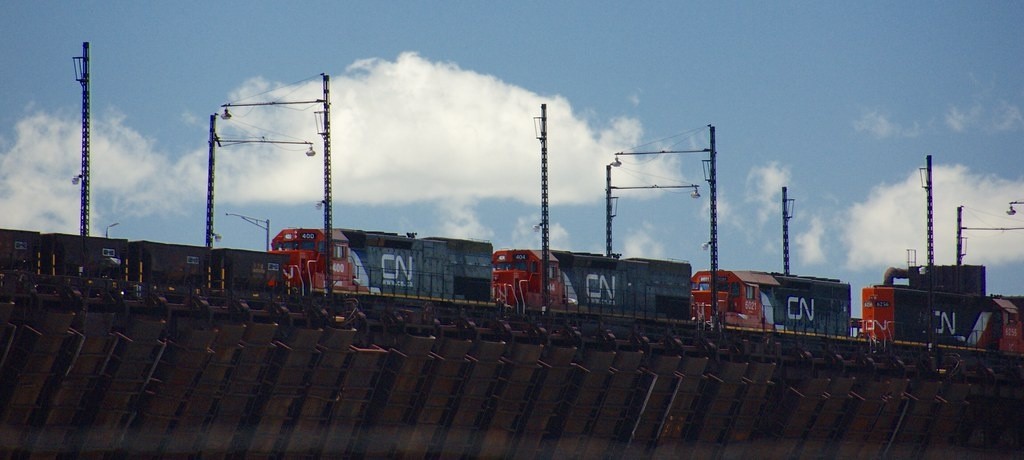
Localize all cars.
[33,284,73,303]
[110,287,126,300]
[67,286,81,298]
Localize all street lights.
[781,185,795,275]
[613,124,720,328]
[956,205,1024,265]
[248,81,255,83]
[604,165,700,261]
[226,213,271,253]
[1007,202,1024,215]
[70,41,91,236]
[919,155,935,265]
[106,222,119,238]
[220,72,335,301]
[206,114,315,251]
[534,103,552,310]
